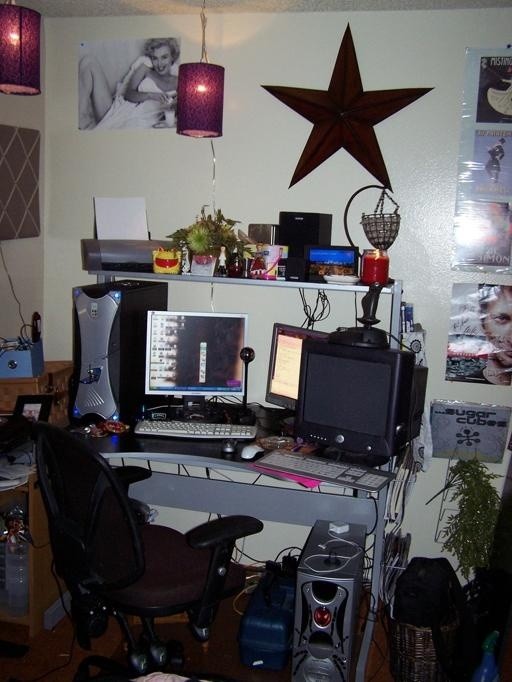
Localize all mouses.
[240,445,264,459]
[222,438,239,453]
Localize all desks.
[42,256,407,682]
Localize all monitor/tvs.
[265,322,331,411]
[294,339,428,467]
[145,310,248,419]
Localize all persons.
[78,37,181,131]
[458,284,512,385]
[484,137,506,184]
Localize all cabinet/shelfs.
[0,474,68,640]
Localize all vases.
[186,246,223,276]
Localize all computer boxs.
[290,520,367,682]
[68,279,168,427]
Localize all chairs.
[31,416,265,681]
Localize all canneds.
[361,249,389,285]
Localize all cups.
[152,250,181,274]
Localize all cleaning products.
[472,630,501,682]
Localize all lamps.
[0,0,42,97]
[171,2,225,141]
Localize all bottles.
[228,253,242,278]
[242,257,251,278]
[30,311,41,344]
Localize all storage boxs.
[0,337,44,378]
[0,360,72,429]
[238,565,294,670]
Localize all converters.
[282,557,295,578]
[328,521,349,534]
[266,560,282,580]
[31,311,41,343]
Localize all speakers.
[279,212,333,280]
[248,224,278,246]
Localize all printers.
[81,221,176,273]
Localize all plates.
[323,274,360,285]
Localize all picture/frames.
[303,244,360,285]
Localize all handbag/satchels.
[393,556,463,628]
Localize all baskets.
[387,596,463,682]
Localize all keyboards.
[254,449,397,492]
[134,420,257,439]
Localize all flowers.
[167,204,251,251]
